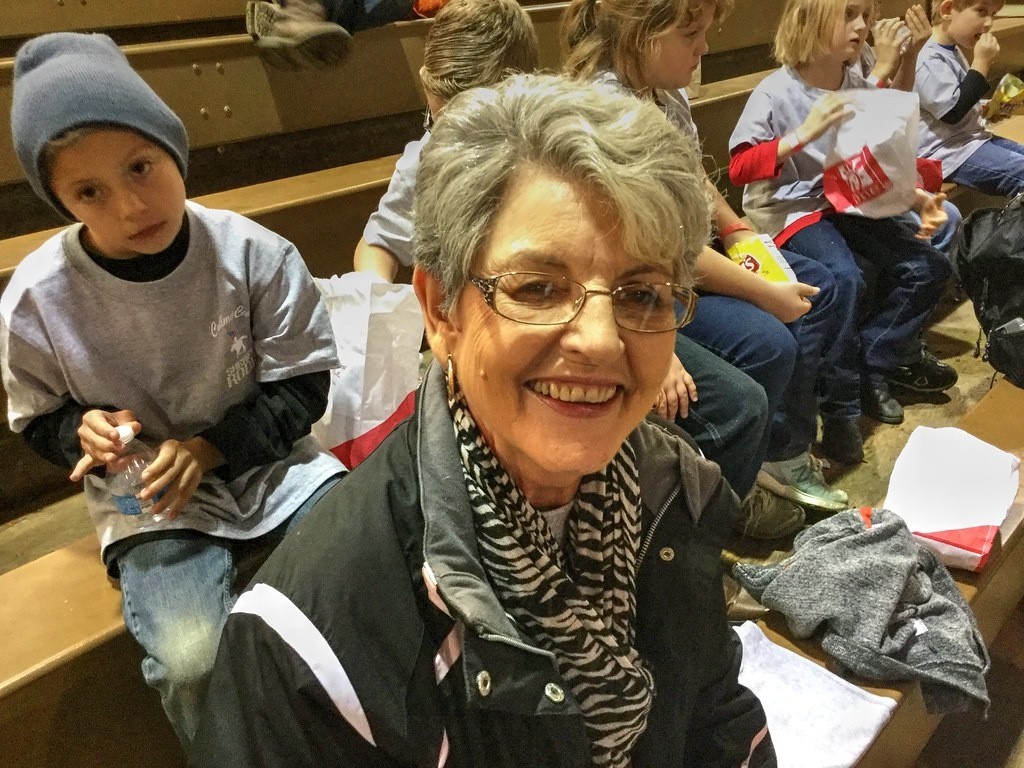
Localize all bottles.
[103,425,173,530]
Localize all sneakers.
[822,420,865,462]
[860,389,904,424]
[245,0,352,71]
[890,352,958,393]
[755,452,849,511]
[732,485,805,540]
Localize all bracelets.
[864,76,885,88]
[718,223,752,245]
[785,130,802,153]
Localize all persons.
[910,1,1024,200]
[847,4,962,392]
[728,0,949,460]
[186,65,781,768]
[0,28,352,747]
[555,1,851,514]
[353,1,806,540]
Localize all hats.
[11,33,188,225]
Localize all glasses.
[462,270,699,333]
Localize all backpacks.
[957,186,1024,383]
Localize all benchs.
[0,0,1022,767]
